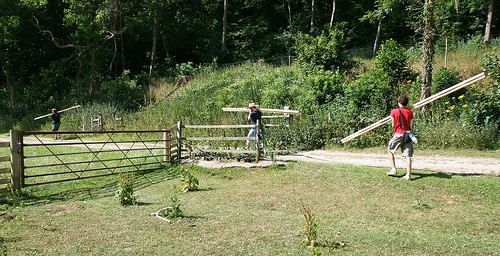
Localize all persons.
[50,109,61,140]
[387,95,413,180]
[247,103,264,148]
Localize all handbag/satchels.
[403,131,418,144]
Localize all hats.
[248,102,260,108]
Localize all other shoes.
[402,174,412,180]
[388,167,398,175]
[54,137,61,140]
